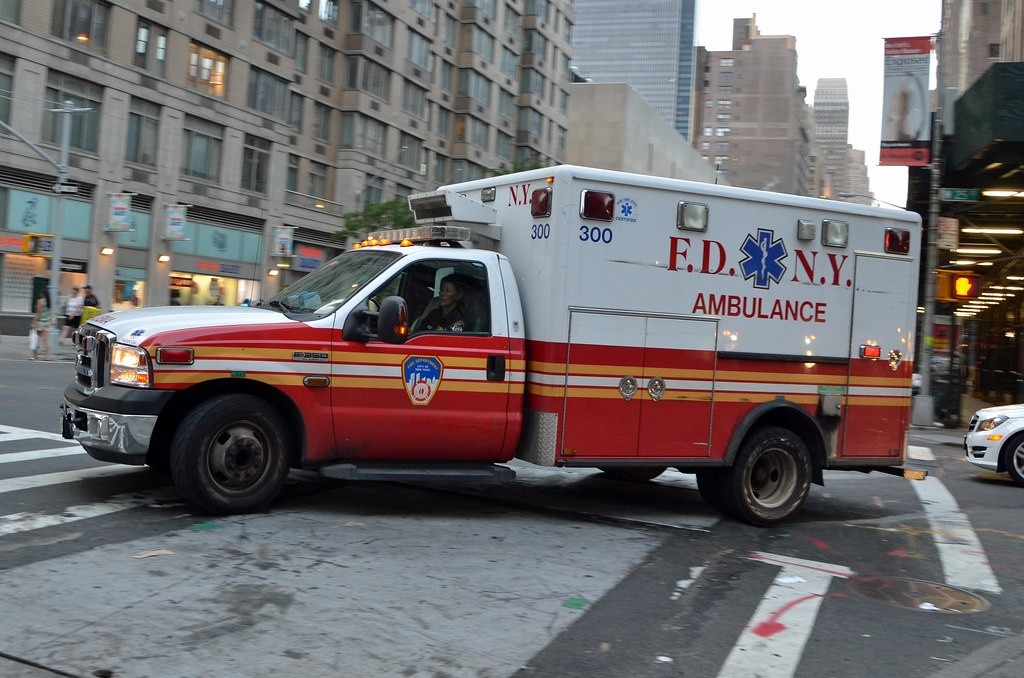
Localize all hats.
[82,285,93,289]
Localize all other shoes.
[29,356,38,360]
[42,356,52,360]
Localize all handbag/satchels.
[28,330,40,350]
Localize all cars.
[962,399,1024,488]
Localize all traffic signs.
[51,182,78,195]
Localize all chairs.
[456,289,488,332]
[409,297,440,333]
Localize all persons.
[58,286,84,343]
[30,298,52,361]
[415,274,472,332]
[82,285,99,307]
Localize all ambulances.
[59,164,923,528]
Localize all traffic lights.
[935,268,985,303]
[23,233,39,254]
